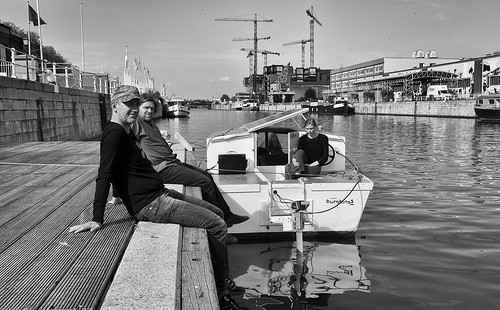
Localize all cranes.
[305,5,323,67]
[233,35,280,76]
[282,38,310,69]
[215,13,282,99]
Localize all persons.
[285,118,329,180]
[129,94,250,226]
[69,84,245,294]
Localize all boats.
[472,87,500,123]
[205,106,375,237]
[167,92,192,118]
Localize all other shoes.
[225,276,246,294]
[224,214,250,228]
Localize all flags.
[29,5,46,26]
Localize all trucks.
[428,85,452,100]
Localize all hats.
[111,85,144,102]
